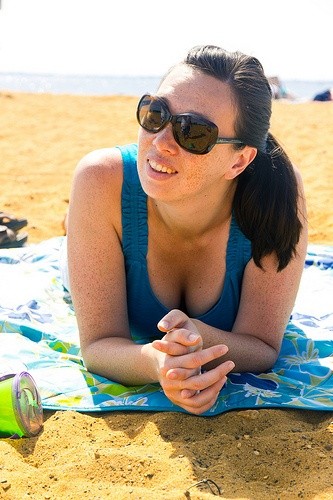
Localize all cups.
[0,370,44,439]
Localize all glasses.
[135,94,245,156]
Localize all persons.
[62,45,308,415]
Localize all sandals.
[0,226,28,250]
[0,212,27,232]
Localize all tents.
[267,76,293,99]
